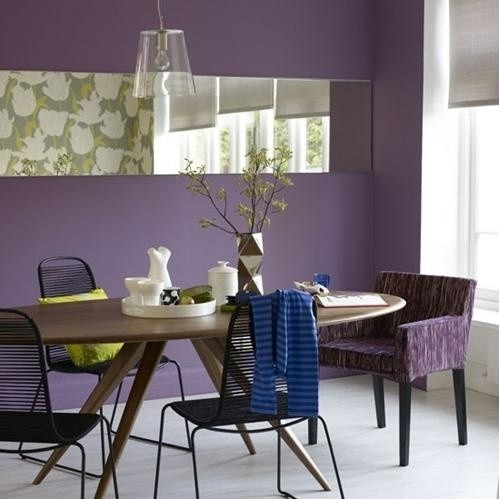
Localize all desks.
[0,288,407,499]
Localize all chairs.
[152,291,345,499]
[18,256,200,499]
[0,306,120,499]
[307,271,478,466]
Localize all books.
[315,293,389,308]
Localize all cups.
[314,273,330,290]
[124,275,182,308]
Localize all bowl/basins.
[179,286,212,305]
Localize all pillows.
[37,289,124,367]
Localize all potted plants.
[179,141,294,299]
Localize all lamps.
[133,0,196,98]
